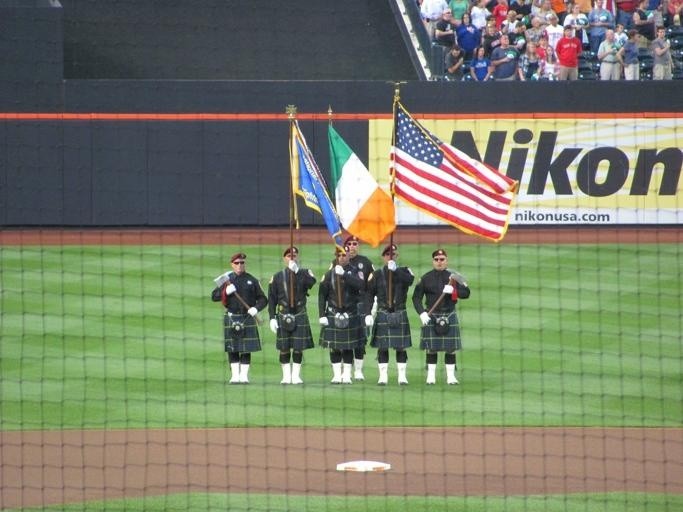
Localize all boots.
[291,361,304,385]
[331,362,342,384]
[354,359,365,381]
[377,362,389,385]
[396,362,408,384]
[240,363,249,384]
[280,362,291,384]
[426,362,437,385]
[342,363,353,384]
[446,363,458,385]
[230,361,239,384]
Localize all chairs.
[462,14,683,83]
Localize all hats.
[346,236,359,241]
[230,252,246,262]
[382,244,398,256]
[337,245,350,253]
[432,248,447,257]
[283,247,298,257]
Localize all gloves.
[225,284,236,295]
[248,307,257,318]
[270,318,278,335]
[288,260,299,274]
[388,259,397,271]
[419,311,431,326]
[442,284,454,294]
[335,265,345,275]
[319,316,329,326]
[365,314,375,326]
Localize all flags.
[287,115,347,257]
[325,122,396,250]
[388,96,519,244]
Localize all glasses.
[346,243,357,246]
[338,254,348,257]
[434,258,446,262]
[287,255,297,258]
[233,261,245,265]
[385,252,396,256]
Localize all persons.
[317,244,365,384]
[268,245,317,385]
[327,235,376,380]
[412,249,470,386]
[416,0,683,82]
[212,251,269,384]
[363,243,415,386]
